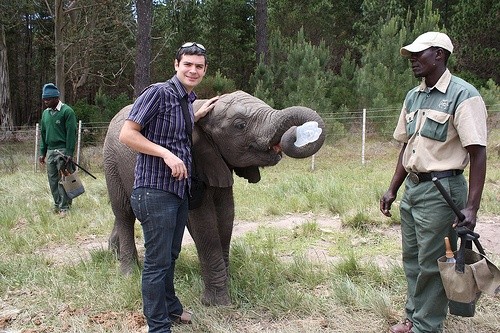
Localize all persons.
[380,31,500,333]
[39,83,97,214]
[119,42,219,332]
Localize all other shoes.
[52,207,70,214]
[169,311,197,326]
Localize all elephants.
[103,90,326,307]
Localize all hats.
[42,83,60,98]
[400,32,453,57]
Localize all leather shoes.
[390,318,421,333]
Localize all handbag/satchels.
[437,226,500,318]
[188,176,207,210]
[58,156,86,199]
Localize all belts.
[409,169,464,184]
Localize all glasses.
[175,42,206,58]
